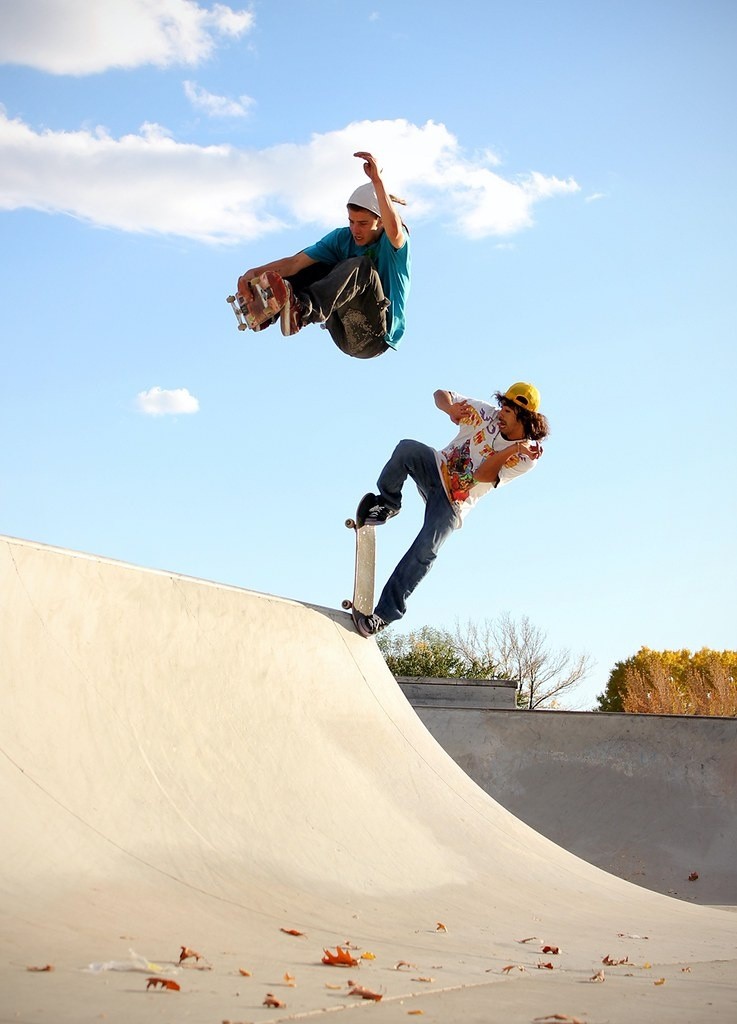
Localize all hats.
[347,180,382,217]
[504,382,540,413]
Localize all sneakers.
[358,613,390,637]
[237,275,270,332]
[364,502,400,525]
[280,280,303,336]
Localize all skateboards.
[225,270,290,332]
[342,492,377,638]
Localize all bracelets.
[516,441,519,453]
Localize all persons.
[351,381,549,638]
[237,151,412,360]
